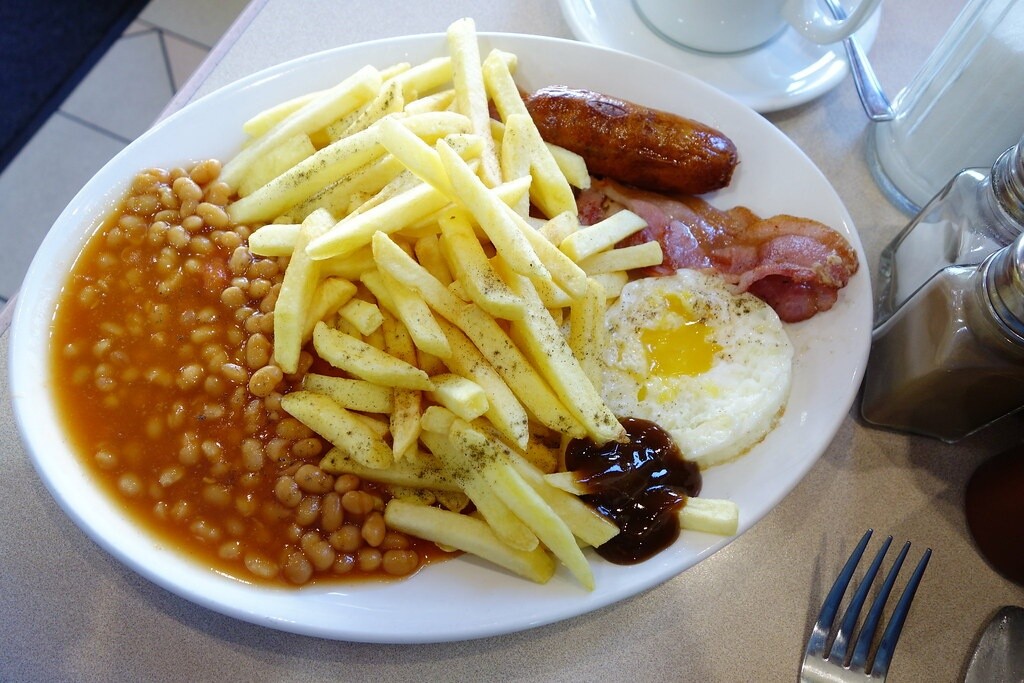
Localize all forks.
[799,527,933,683]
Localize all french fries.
[210,17,740,589]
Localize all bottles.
[861,232,1024,443]
[869,137,1024,329]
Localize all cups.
[632,1,880,55]
[867,0,1024,217]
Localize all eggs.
[591,267,793,472]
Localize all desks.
[0,0,1024,683]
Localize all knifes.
[963,604,1024,683]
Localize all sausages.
[529,85,738,197]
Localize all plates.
[558,0,882,114]
[7,31,874,645]
